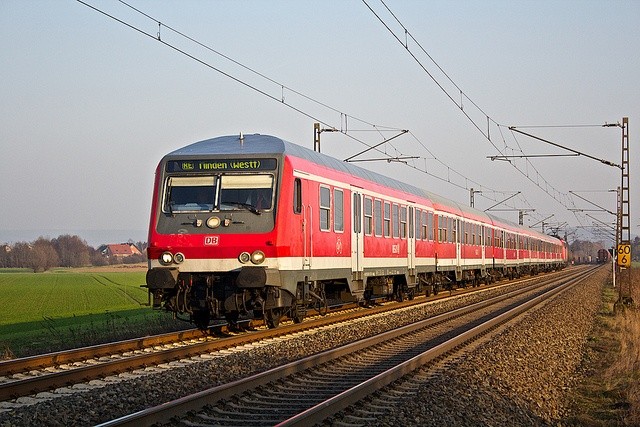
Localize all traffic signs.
[618,245,631,266]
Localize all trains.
[598,248,612,261]
[140,132,568,329]
